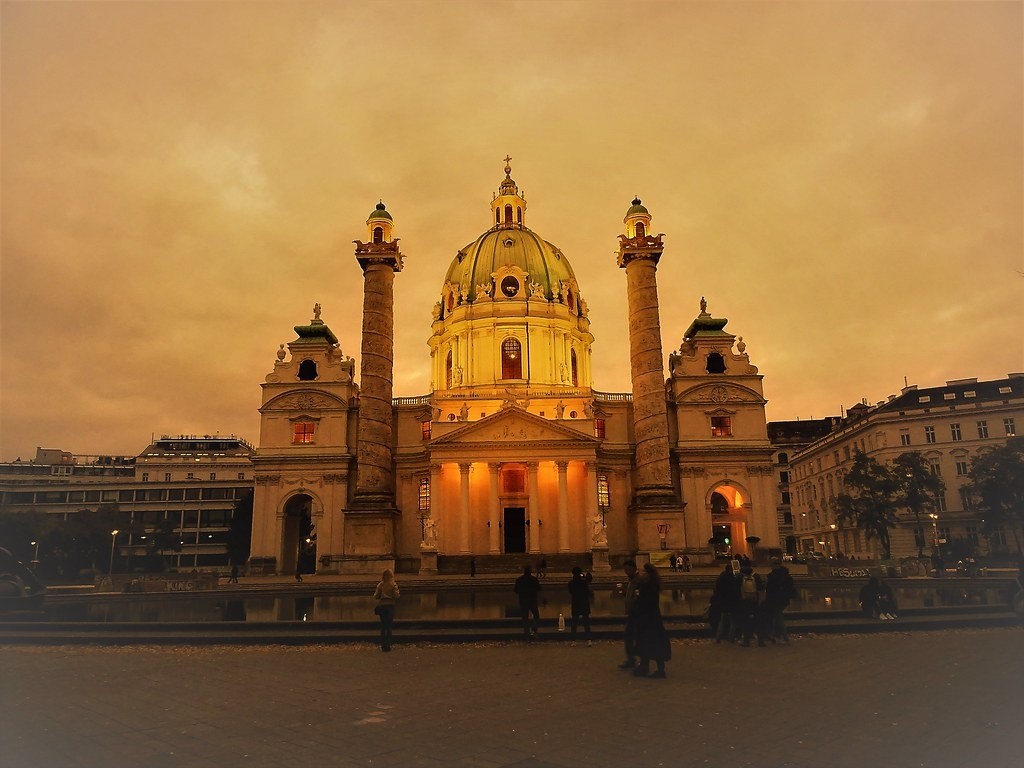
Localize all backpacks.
[739,570,760,604]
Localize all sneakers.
[879,611,894,621]
[585,640,592,647]
[530,631,538,639]
[570,640,576,647]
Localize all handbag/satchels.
[374,606,380,616]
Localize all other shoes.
[382,644,391,652]
[780,638,790,645]
[649,670,665,678]
[632,668,648,676]
[741,642,749,647]
[618,661,635,668]
[891,612,898,619]
[715,639,722,645]
[728,638,736,644]
[758,642,766,647]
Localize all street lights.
[929,513,941,559]
[108,529,118,577]
[31,541,39,569]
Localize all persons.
[471,557,476,577]
[514,567,542,642]
[232,565,238,583]
[373,569,400,652]
[568,566,593,647]
[710,552,797,648]
[616,561,671,679]
[296,560,303,582]
[535,556,547,579]
[858,577,897,621]
[669,552,692,572]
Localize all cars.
[783,551,825,561]
